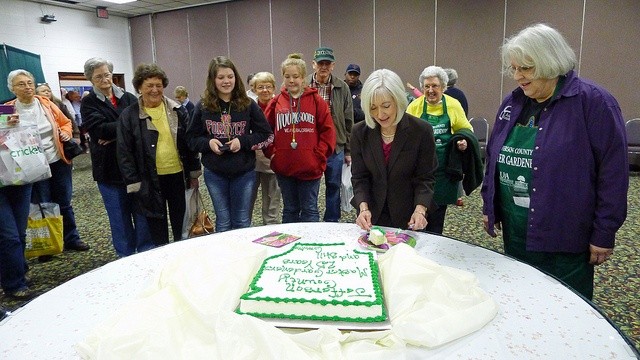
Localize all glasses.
[508,65,535,74]
[35,82,48,87]
[422,83,442,89]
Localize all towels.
[371,240,500,348]
[282,326,405,360]
[77,285,310,360]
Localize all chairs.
[466,114,488,171]
[617,116,639,175]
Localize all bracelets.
[413,210,425,217]
[359,208,369,213]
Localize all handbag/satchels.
[57,123,84,160]
[188,188,214,238]
[24,189,64,259]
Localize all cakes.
[232,242,389,323]
[368,226,388,246]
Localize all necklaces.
[290,101,298,150]
[144,106,164,120]
[291,94,298,108]
[381,132,396,138]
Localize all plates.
[354,229,422,253]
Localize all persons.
[65,89,88,154]
[261,54,336,224]
[35,82,76,133]
[405,65,474,234]
[246,73,276,104]
[174,86,194,116]
[350,68,437,231]
[117,64,202,244]
[1,105,34,299]
[3,69,90,263]
[249,72,280,224]
[183,55,274,232]
[343,63,365,123]
[406,70,467,116]
[482,24,629,306]
[303,48,351,223]
[80,57,158,259]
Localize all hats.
[174,86,188,97]
[313,47,335,63]
[346,64,360,75]
